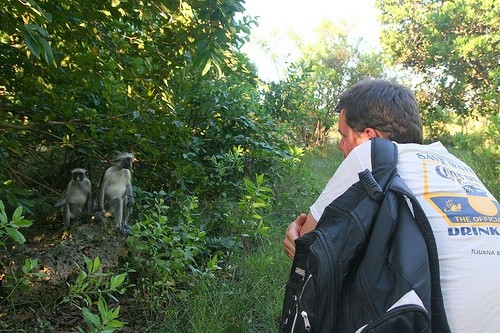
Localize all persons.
[284,80,500,333]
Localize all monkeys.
[95,154,134,235]
[54,168,93,230]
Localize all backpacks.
[280,138,451,333]
